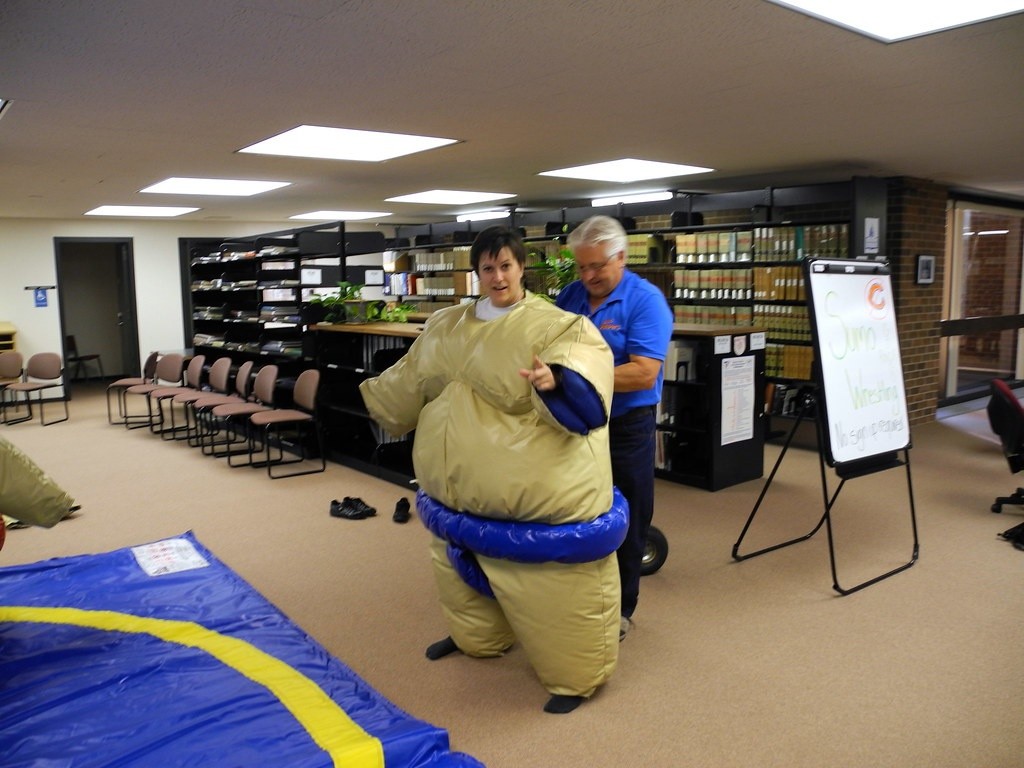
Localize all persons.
[412,226,628,716]
[551,214,675,638]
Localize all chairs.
[0,352,33,424]
[210,365,283,469]
[150,355,209,440]
[2,353,69,427]
[123,353,190,433]
[249,370,325,479]
[190,361,255,455]
[106,352,164,426]
[66,335,104,383]
[170,358,236,448]
[987,378,1024,513]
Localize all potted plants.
[305,279,419,326]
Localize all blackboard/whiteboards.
[803,256,913,467]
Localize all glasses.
[574,253,613,273]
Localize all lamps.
[591,192,673,208]
[456,211,510,222]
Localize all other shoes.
[619,616,630,641]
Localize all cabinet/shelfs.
[188,217,852,492]
[0,322,20,385]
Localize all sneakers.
[393,497,410,523]
[342,497,376,516]
[330,500,366,519]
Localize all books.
[361,333,410,373]
[625,223,850,381]
[189,244,302,359]
[654,425,679,473]
[382,223,573,326]
[377,421,411,445]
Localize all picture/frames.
[914,254,936,284]
